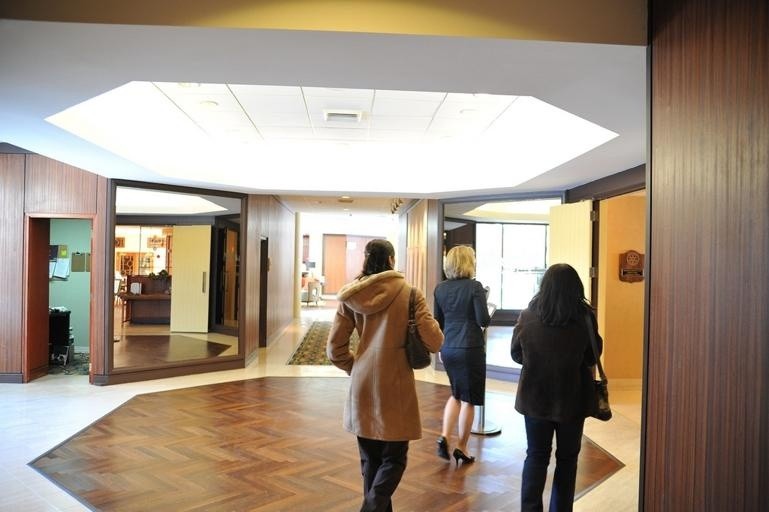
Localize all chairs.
[48,311,73,366]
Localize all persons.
[326,239,444,512]
[510,264,603,512]
[434,245,491,466]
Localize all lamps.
[391,197,404,214]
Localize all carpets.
[285,321,360,365]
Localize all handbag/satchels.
[592,360,612,422]
[405,319,431,369]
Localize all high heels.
[453,448,476,465]
[435,435,451,462]
